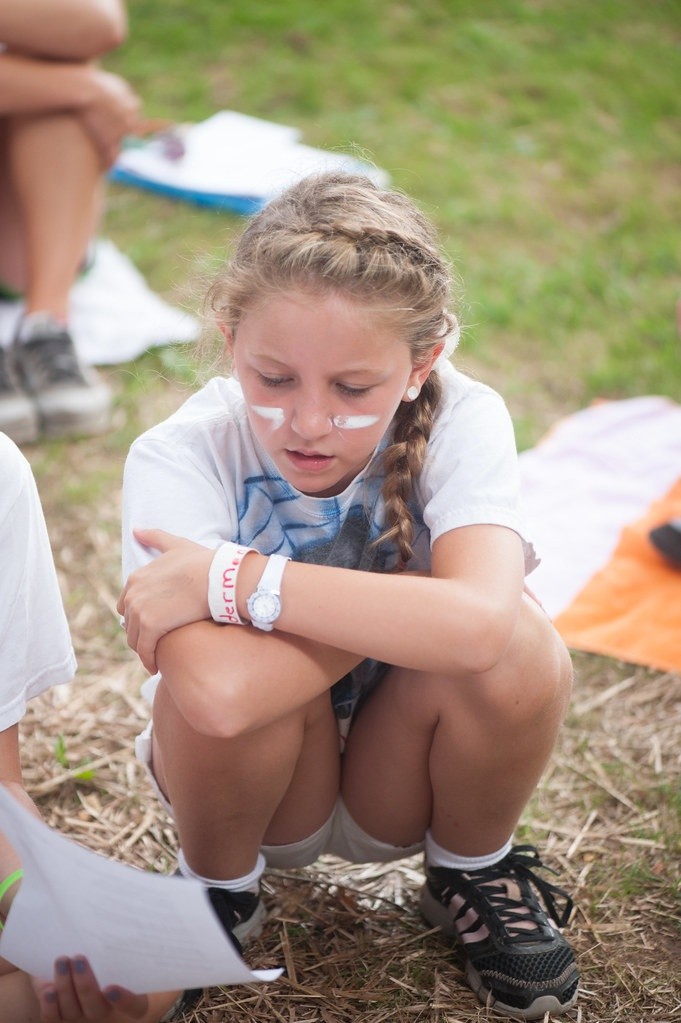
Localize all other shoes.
[17,332,107,439]
[0,342,39,443]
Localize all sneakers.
[160,868,267,1022]
[419,845,597,1020]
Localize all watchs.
[246,554,292,633]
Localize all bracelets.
[207,539,262,628]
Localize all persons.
[115,168,584,1023]
[0,0,144,448]
[0,429,182,1023]
